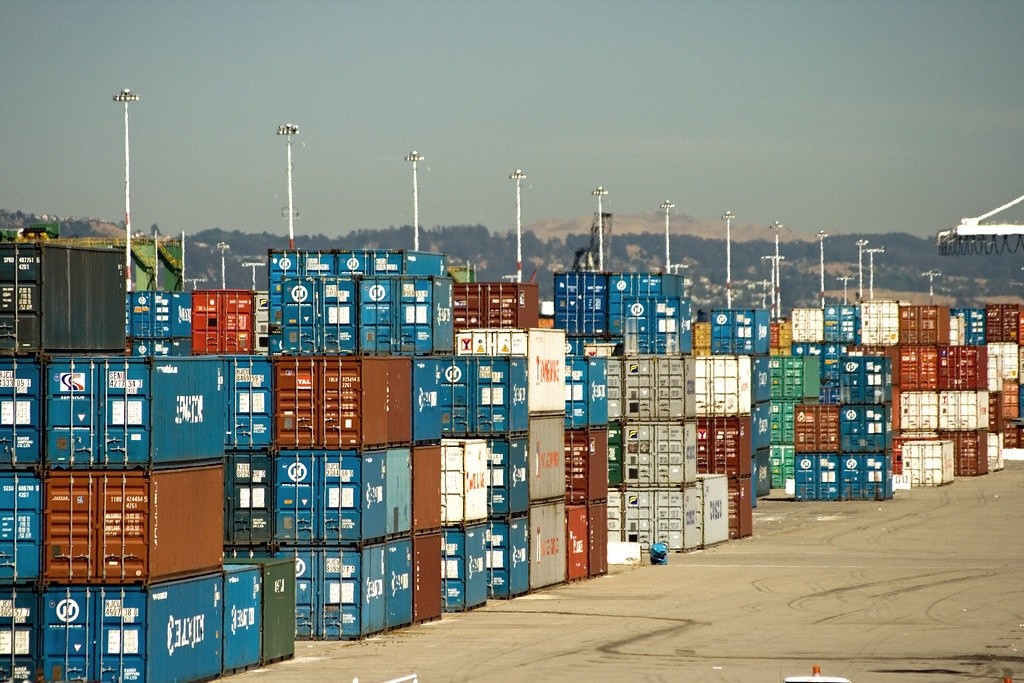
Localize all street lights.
[114,88,142,290]
[242,262,266,290]
[275,120,306,250]
[215,239,228,289]
[922,271,942,306]
[867,248,885,300]
[758,220,786,322]
[660,200,688,276]
[855,239,869,300]
[593,185,609,272]
[815,228,828,309]
[183,277,207,290]
[721,210,735,308]
[404,149,425,252]
[501,168,527,284]
[838,278,855,306]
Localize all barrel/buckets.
[650,541,670,565]
